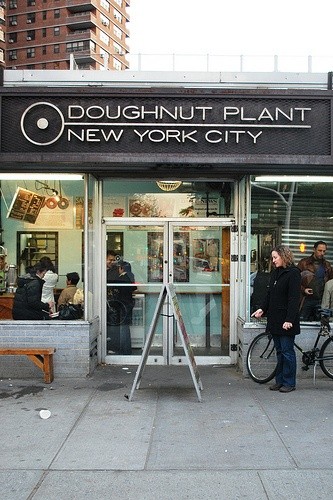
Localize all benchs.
[0,347,56,384]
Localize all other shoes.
[280,385,296,392]
[269,384,281,390]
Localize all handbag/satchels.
[58,304,82,319]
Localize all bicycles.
[246,308,333,384]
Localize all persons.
[57,272,83,311]
[112,262,138,355]
[320,278,333,322]
[106,250,120,351]
[39,256,59,315]
[297,241,333,322]
[251,245,302,393]
[11,262,56,321]
